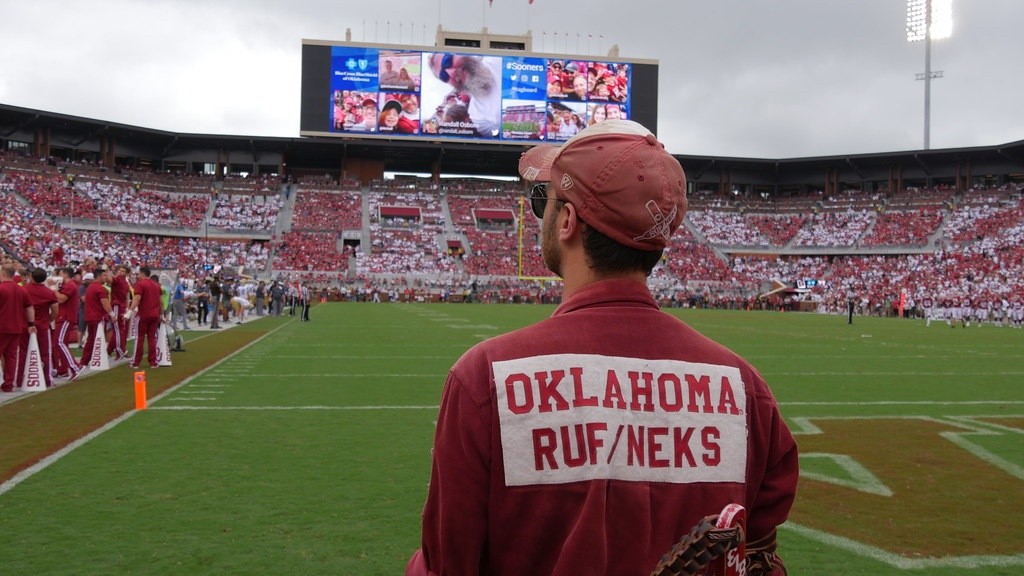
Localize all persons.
[0,119,1024,576]
[333,50,628,142]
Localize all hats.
[383,99,402,116]
[362,99,377,107]
[602,71,614,78]
[344,97,355,103]
[565,63,578,72]
[82,273,94,280]
[552,61,563,68]
[518,120,686,250]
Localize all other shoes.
[151,364,160,368]
[128,364,140,369]
[69,364,86,382]
[115,350,128,361]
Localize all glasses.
[553,66,562,70]
[566,71,574,75]
[529,184,569,219]
[439,54,455,83]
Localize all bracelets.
[109,311,115,318]
[160,314,165,320]
[28,322,35,327]
